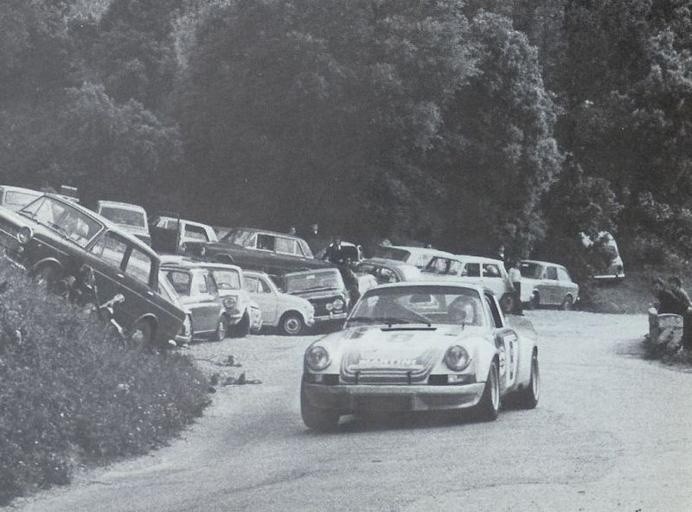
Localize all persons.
[667,275,692,358]
[495,244,509,264]
[508,257,525,317]
[287,221,300,239]
[647,275,679,321]
[319,232,348,272]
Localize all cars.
[572,224,629,286]
[299,283,540,429]
